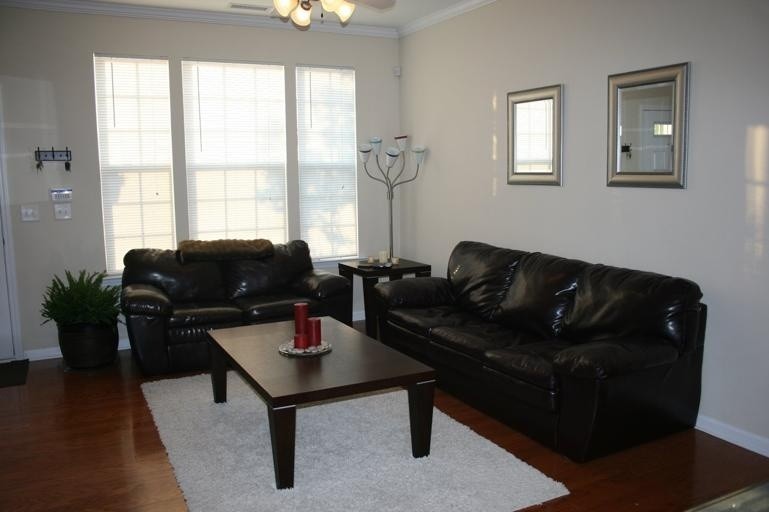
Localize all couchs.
[122,240,350,377]
[371,241,707,466]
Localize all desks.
[336,257,431,341]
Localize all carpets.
[141,370,572,511]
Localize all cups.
[391,256,399,265]
[294,303,309,334]
[308,318,321,346]
[379,251,388,267]
[293,333,309,348]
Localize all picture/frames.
[506,84,564,187]
[605,60,689,189]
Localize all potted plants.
[36,268,125,376]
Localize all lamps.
[358,134,427,258]
[270,0,355,29]
